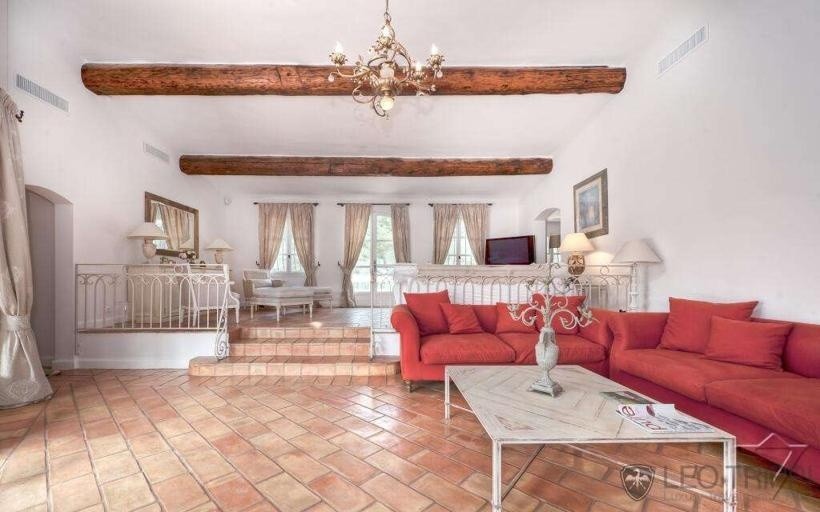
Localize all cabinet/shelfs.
[125,263,241,327]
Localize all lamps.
[204,237,234,264]
[126,221,170,266]
[178,237,195,251]
[325,1,448,119]
[556,232,595,279]
[607,239,664,312]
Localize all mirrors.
[143,191,201,259]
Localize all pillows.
[248,278,273,289]
[401,288,452,338]
[703,311,795,374]
[530,291,588,336]
[439,301,485,335]
[653,295,760,357]
[492,301,541,335]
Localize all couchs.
[598,298,820,492]
[389,285,628,395]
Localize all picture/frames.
[572,167,609,240]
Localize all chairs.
[272,270,334,316]
[242,268,273,311]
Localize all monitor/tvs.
[485,236,534,264]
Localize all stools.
[250,286,315,323]
